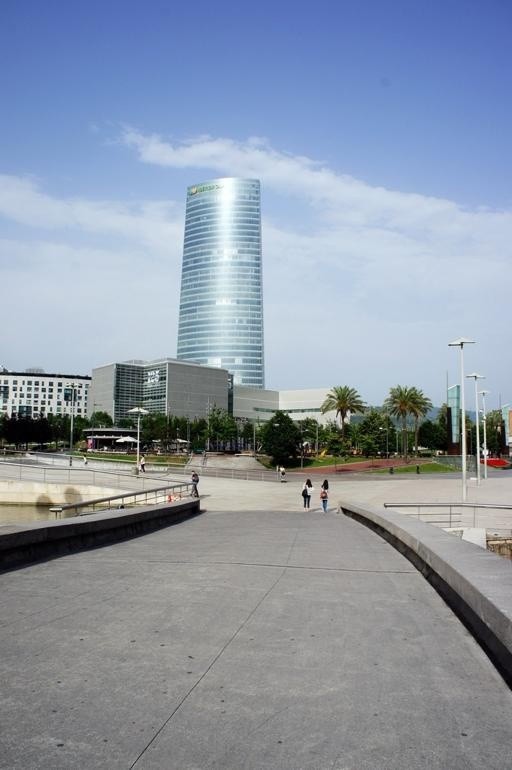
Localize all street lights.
[465,373,485,483]
[127,406,149,475]
[379,426,410,463]
[477,390,489,464]
[447,336,477,503]
[306,422,332,456]
[64,382,80,466]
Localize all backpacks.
[301,489,308,498]
[320,491,327,498]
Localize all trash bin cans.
[416,465,420,474]
[279,467,288,482]
[132,467,139,475]
[389,467,393,474]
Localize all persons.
[190,470,199,497]
[320,480,329,514]
[279,464,285,478]
[141,455,146,472]
[281,472,287,481]
[301,479,313,512]
[84,455,87,464]
